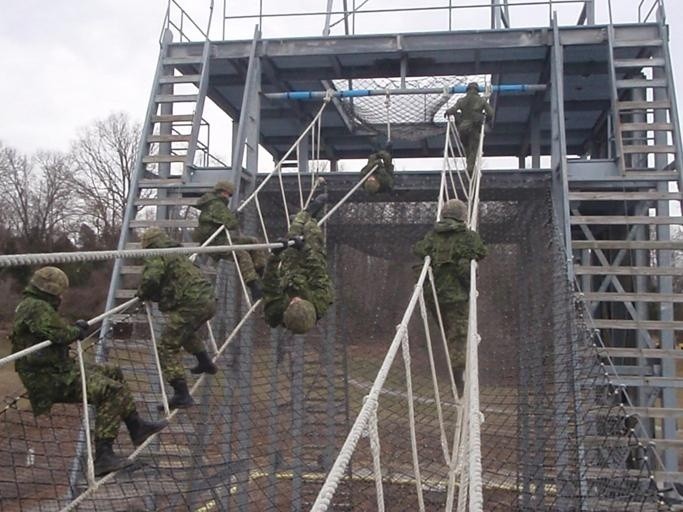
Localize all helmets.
[212,179,236,196]
[364,179,381,195]
[439,197,469,222]
[465,82,480,92]
[281,299,318,335]
[29,265,70,299]
[141,227,167,251]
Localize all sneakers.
[384,141,394,155]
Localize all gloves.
[75,318,89,342]
[289,234,303,251]
[270,237,289,256]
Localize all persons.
[412,198,488,400]
[6,267,167,478]
[261,194,335,332]
[359,139,397,196]
[443,81,495,179]
[135,223,220,409]
[195,182,264,302]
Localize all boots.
[306,193,331,218]
[122,414,170,445]
[191,351,219,375]
[158,377,196,410]
[92,437,135,477]
[247,281,268,300]
[288,214,298,223]
